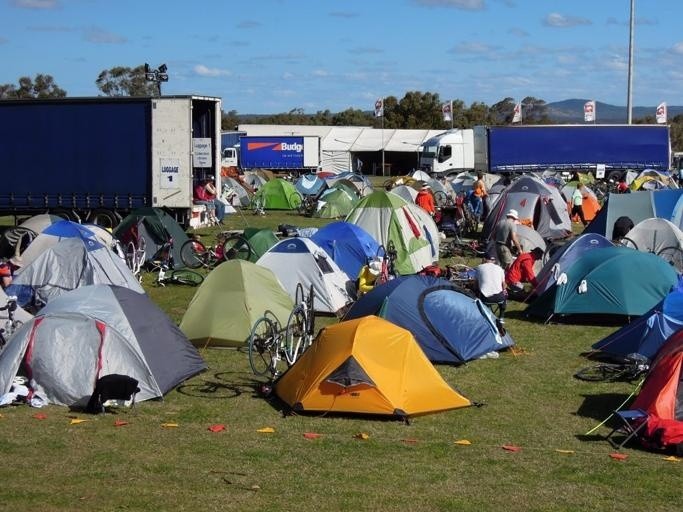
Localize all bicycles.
[609,234,683,276]
[247,283,317,394]
[382,178,417,193]
[250,191,265,216]
[0,294,28,358]
[582,178,633,202]
[577,353,656,384]
[540,239,570,268]
[123,231,251,290]
[286,191,317,214]
[432,190,491,292]
[377,240,400,282]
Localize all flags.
[441,100,454,123]
[582,99,596,122]
[654,101,668,124]
[511,99,523,124]
[373,95,384,118]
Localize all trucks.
[220,136,320,179]
[0,94,222,236]
[417,125,671,185]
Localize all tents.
[1,163,683,455]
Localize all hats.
[530,247,544,260]
[206,175,215,181]
[506,209,519,220]
[484,252,496,260]
[421,183,431,189]
[9,256,24,268]
[369,256,385,275]
